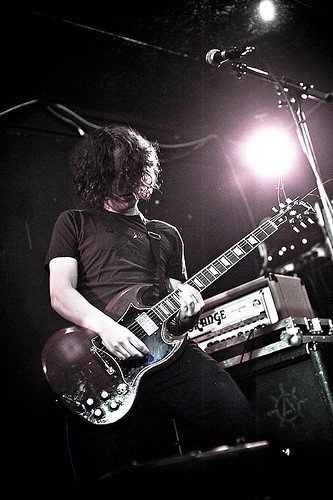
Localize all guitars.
[41,195,315,426]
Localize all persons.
[43,127,252,478]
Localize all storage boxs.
[172,342,333,500]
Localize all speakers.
[86,440,294,500]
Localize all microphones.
[205,46,255,67]
